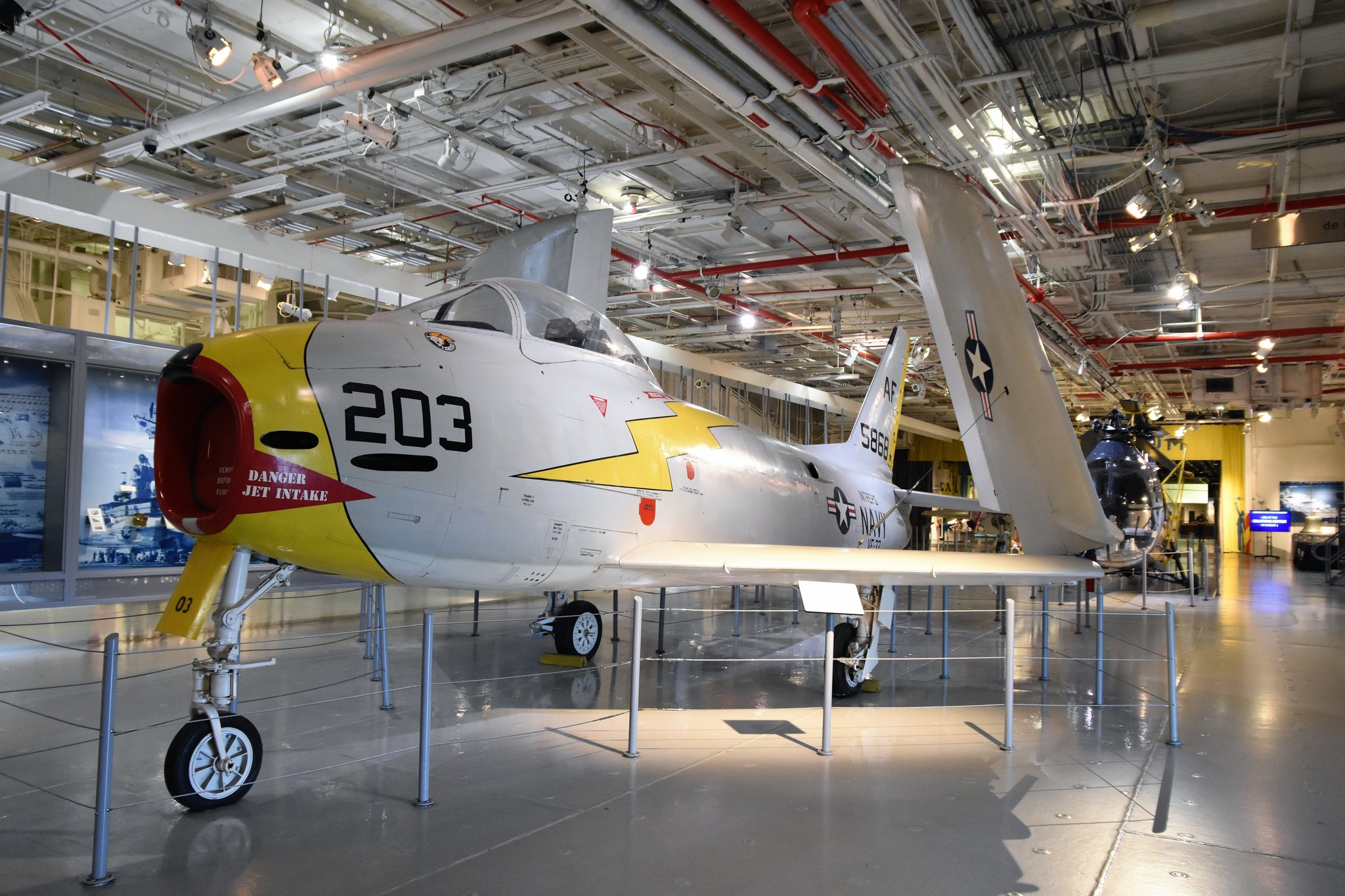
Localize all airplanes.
[151,205,1106,813]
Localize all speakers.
[1326,422,1345,446]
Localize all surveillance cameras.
[142,139,158,154]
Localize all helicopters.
[1078,397,1200,599]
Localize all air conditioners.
[168,251,186,267]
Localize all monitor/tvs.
[1249,510,1291,532]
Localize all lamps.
[844,343,862,366]
[256,273,275,291]
[620,189,647,214]
[186,1,289,92]
[1077,153,1275,424]
[634,240,656,280]
[912,341,930,367]
[341,95,460,170]
[200,259,214,284]
[321,288,338,302]
[695,379,711,389]
